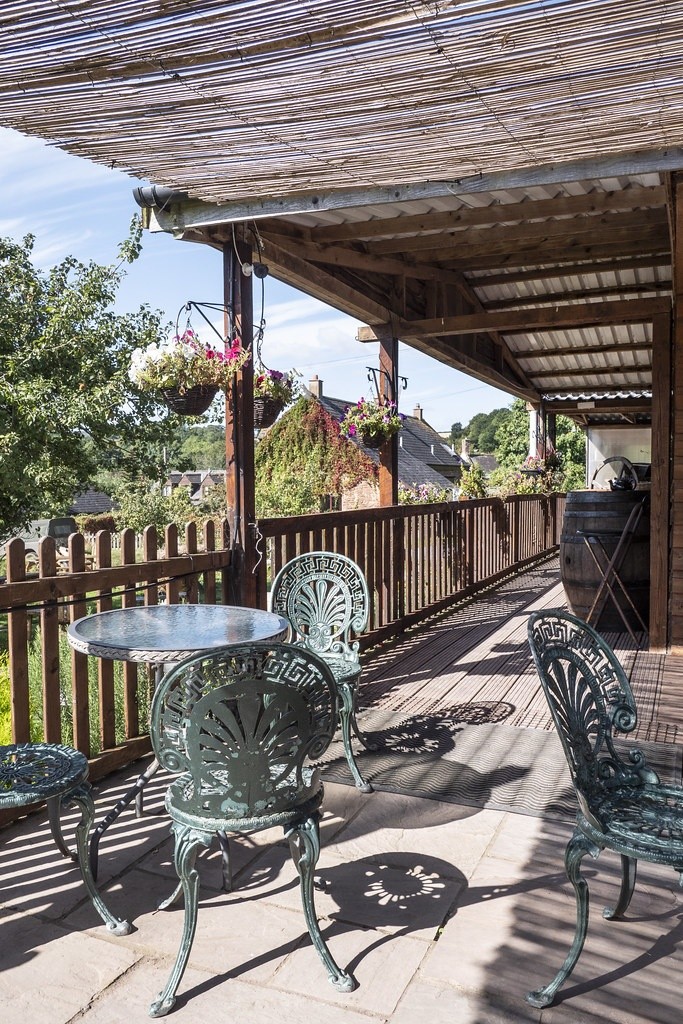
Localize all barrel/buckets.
[560,492,650,631]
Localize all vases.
[253,397,284,428]
[158,385,222,415]
[519,470,543,479]
[361,429,386,449]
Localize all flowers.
[339,397,406,437]
[129,330,254,397]
[252,368,305,403]
[524,456,546,472]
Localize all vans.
[5,517,76,561]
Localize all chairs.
[268,551,377,793]
[0,743,132,937]
[523,608,683,1010]
[148,642,359,1019]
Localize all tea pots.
[608,478,636,491]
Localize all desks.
[66,603,289,893]
